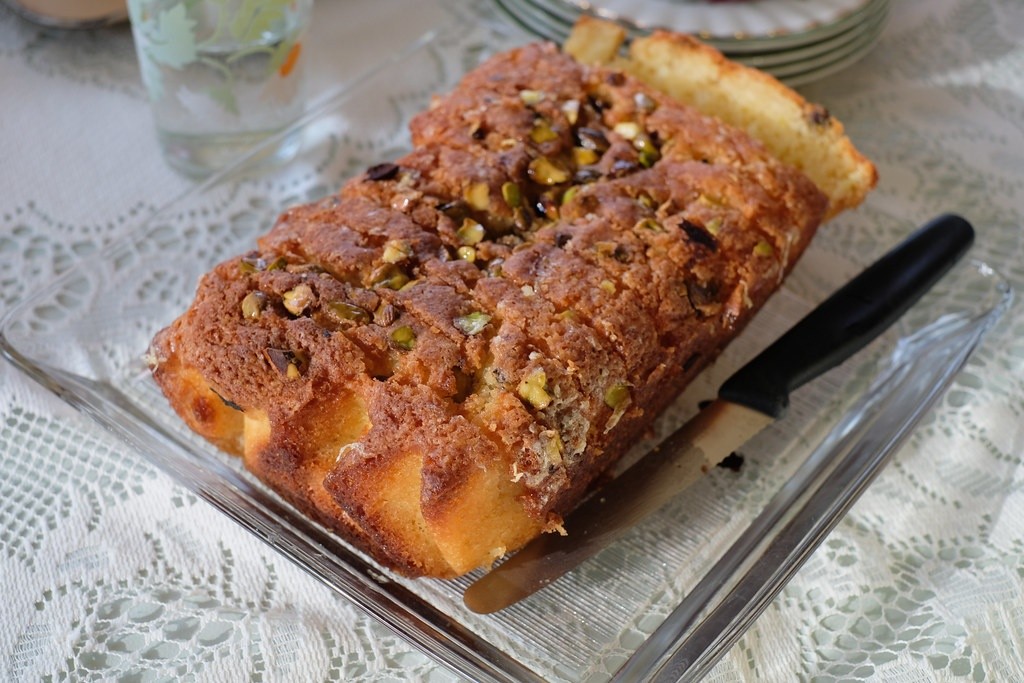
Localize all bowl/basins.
[0,0,132,30]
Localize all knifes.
[461,213,976,614]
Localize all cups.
[126,0,313,183]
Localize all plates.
[487,1,890,91]
[0,10,1018,683]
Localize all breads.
[143,40,829,577]
[561,15,879,223]
